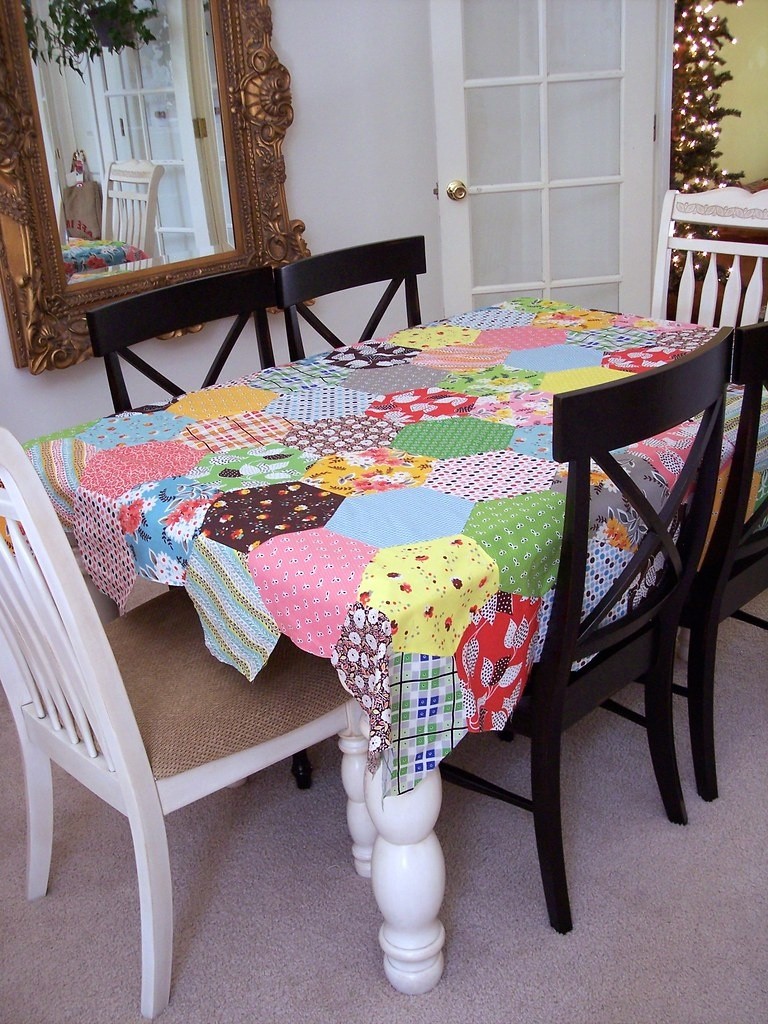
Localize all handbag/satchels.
[62,151,103,240]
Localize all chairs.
[274,233,427,363]
[0,429,379,1019]
[632,320,768,803]
[651,186,768,329]
[438,326,733,934]
[84,263,316,792]
[100,158,165,259]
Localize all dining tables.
[61,238,150,284]
[0,298,768,995]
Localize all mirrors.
[1,0,316,376]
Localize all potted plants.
[22,0,160,83]
